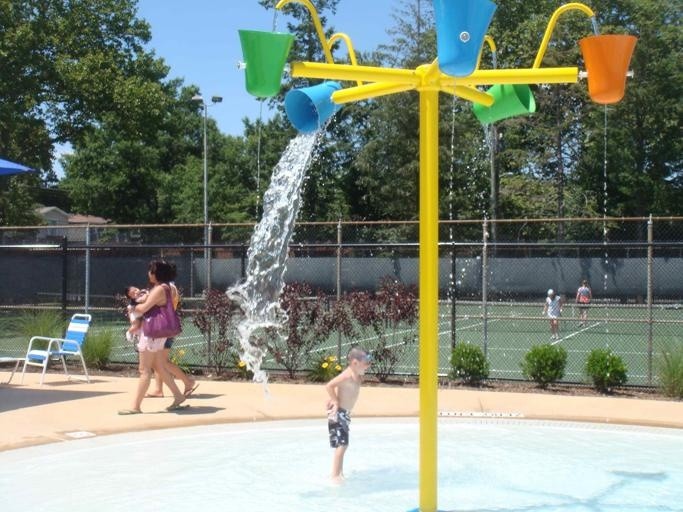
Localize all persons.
[118,259,200,415]
[324,345,372,485]
[542,289,563,340]
[576,279,592,327]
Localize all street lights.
[188,93,226,297]
[254,96,269,221]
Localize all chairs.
[20,313,92,386]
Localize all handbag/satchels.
[142,286,182,338]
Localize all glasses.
[355,355,374,362]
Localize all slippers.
[118,408,143,415]
[144,392,165,398]
[166,383,200,411]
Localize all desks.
[0,357,28,384]
